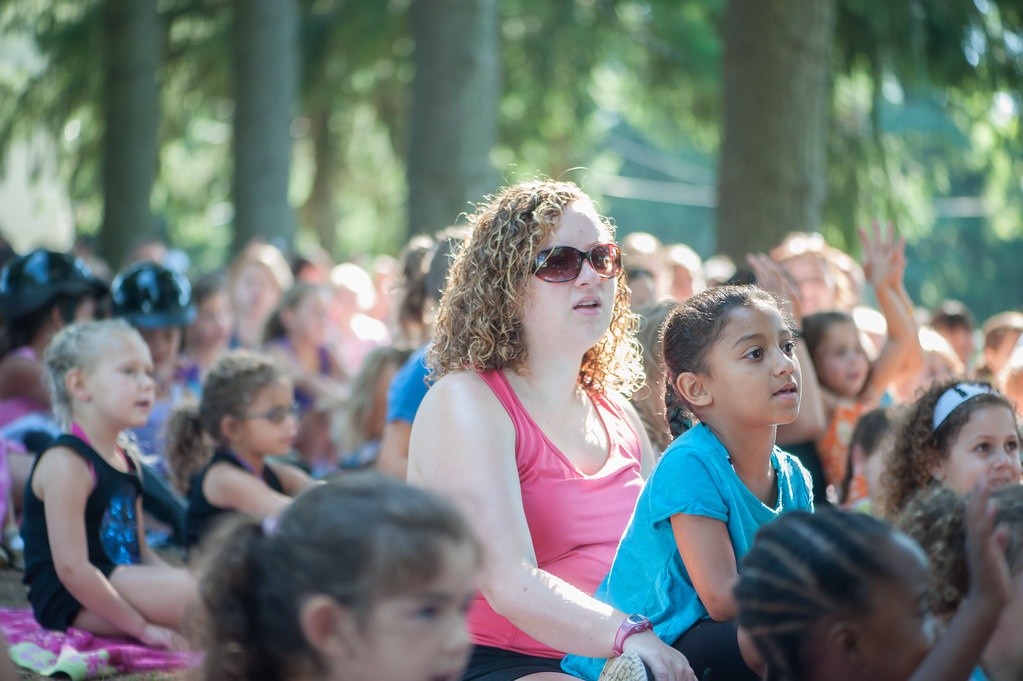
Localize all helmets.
[0,248,109,330]
[111,261,197,332]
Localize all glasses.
[517,243,622,282]
[234,401,299,425]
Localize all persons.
[0,232,1023,600]
[407,179,698,681]
[733,481,1023,681]
[607,285,813,681]
[23,322,210,656]
[197,477,479,680]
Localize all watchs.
[613,613,654,655]
